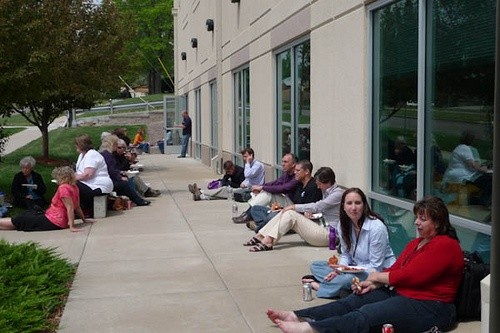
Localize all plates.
[336,266,368,273]
[22,184,37,187]
[310,213,322,219]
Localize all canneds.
[303,282,312,301]
[127,200,132,210]
[381,323,394,333]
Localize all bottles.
[227,185,232,204]
[232,202,238,217]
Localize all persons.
[72,133,115,219]
[246,156,323,233]
[241,165,345,253]
[10,154,48,214]
[187,147,266,201]
[0,165,98,232]
[221,159,246,188]
[280,126,310,158]
[441,127,493,211]
[393,127,445,201]
[267,194,464,333]
[382,137,416,196]
[300,186,398,299]
[95,125,161,208]
[157,125,173,154]
[230,152,303,224]
[177,109,192,158]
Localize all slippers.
[244,238,273,252]
[301,276,320,288]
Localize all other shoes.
[136,198,150,205]
[188,183,201,200]
[144,187,162,197]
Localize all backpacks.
[458,251,489,321]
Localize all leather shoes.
[233,212,250,222]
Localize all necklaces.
[401,237,427,267]
[350,219,365,258]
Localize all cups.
[329,228,336,250]
